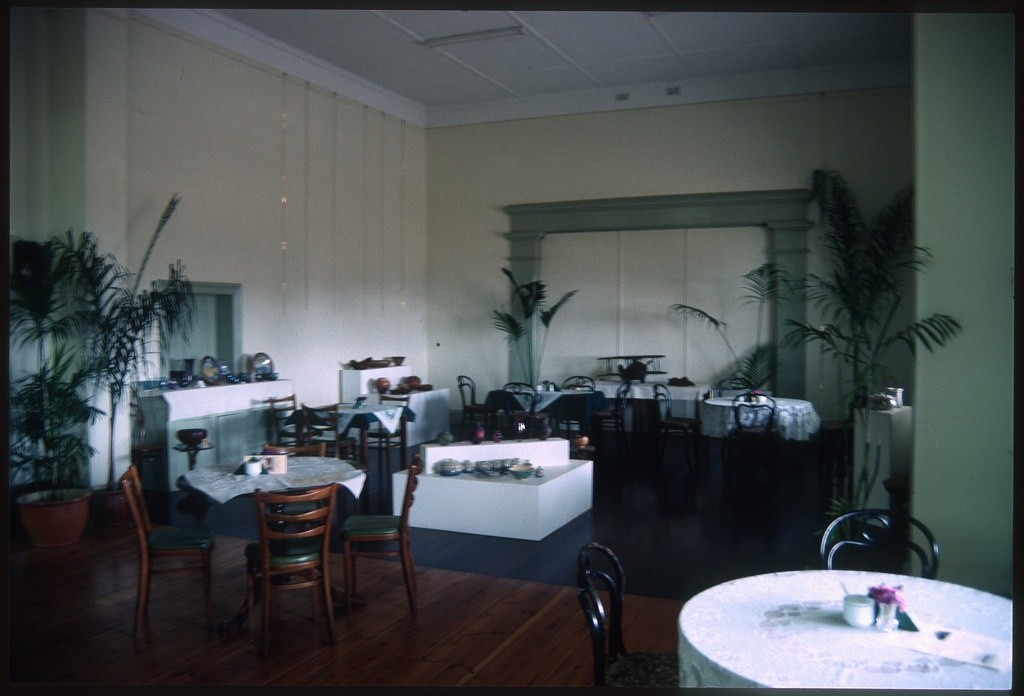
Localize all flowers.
[870,584,906,613]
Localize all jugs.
[434,458,543,477]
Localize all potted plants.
[5,299,108,548]
[14,191,199,531]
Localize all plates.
[252,352,273,374]
[217,361,231,376]
[200,356,221,386]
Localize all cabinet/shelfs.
[138,377,297,494]
[339,366,451,450]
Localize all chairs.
[821,509,939,580]
[457,374,596,441]
[817,387,868,482]
[245,486,338,658]
[652,383,703,474]
[120,465,215,639]
[714,378,776,476]
[267,395,412,483]
[339,454,423,622]
[590,382,631,458]
[577,543,681,686]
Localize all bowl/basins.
[507,466,533,480]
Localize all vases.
[876,602,899,632]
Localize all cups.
[245,463,262,477]
[844,594,875,628]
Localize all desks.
[283,402,415,512]
[486,388,606,435]
[578,380,710,431]
[679,569,1013,691]
[700,396,822,443]
[175,455,366,639]
